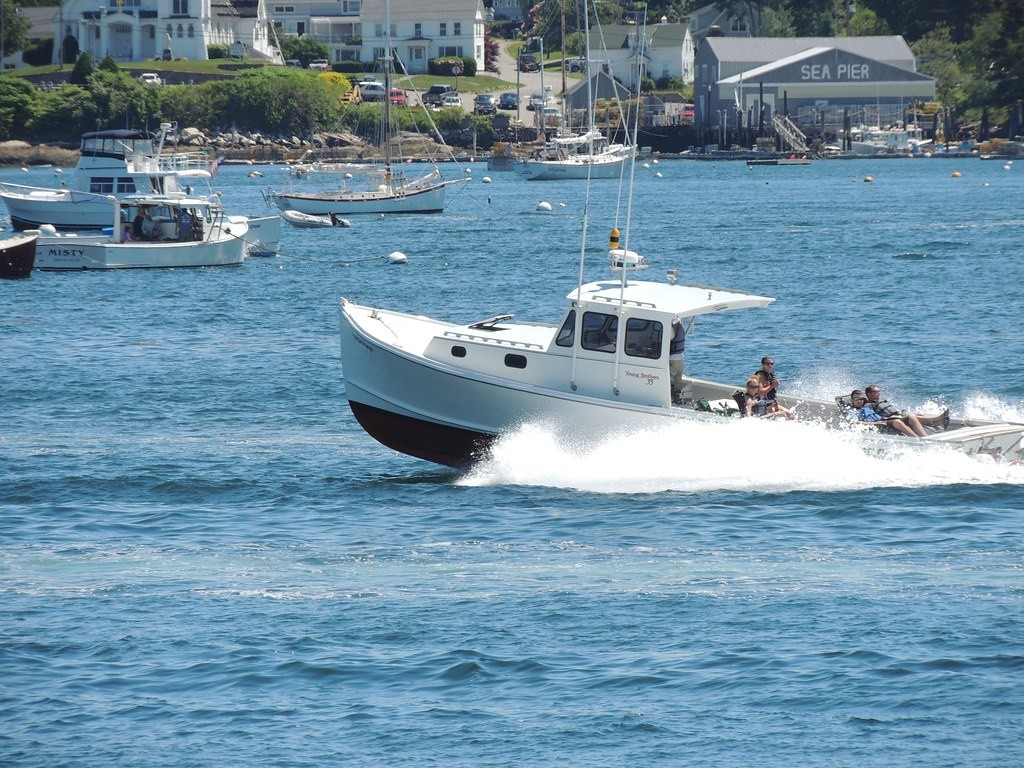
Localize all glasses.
[748,385,759,389]
[853,397,863,401]
[763,363,774,365]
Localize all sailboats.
[486,0,639,178]
[273,0,473,211]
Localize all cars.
[308,59,332,70]
[517,54,540,72]
[441,97,463,108]
[140,72,162,86]
[540,107,561,124]
[500,93,519,108]
[473,95,499,114]
[680,105,695,120]
[568,57,587,72]
[528,93,548,107]
[389,88,410,107]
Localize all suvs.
[359,81,396,97]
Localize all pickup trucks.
[422,83,458,103]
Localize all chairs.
[732,389,781,420]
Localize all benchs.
[836,392,888,434]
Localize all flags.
[211,154,225,177]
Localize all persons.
[907,142,918,153]
[744,356,795,421]
[790,153,807,159]
[851,385,951,437]
[963,139,974,152]
[205,191,223,220]
[669,323,687,381]
[123,207,199,242]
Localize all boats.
[1,120,280,279]
[283,210,332,228]
[335,0,1024,475]
